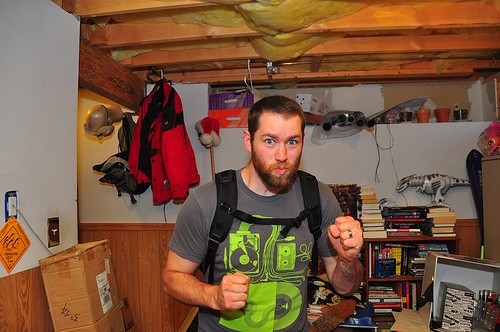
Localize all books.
[307,185,459,332]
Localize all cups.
[461,109,468,120]
[399,112,412,121]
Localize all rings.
[348,229,353,239]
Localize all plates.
[450,119,472,122]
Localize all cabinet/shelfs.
[354,235,460,322]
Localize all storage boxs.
[38,238,139,331]
[295,93,329,116]
[436,281,476,332]
[418,251,500,332]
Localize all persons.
[164,95,365,332]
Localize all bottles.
[453,105,460,120]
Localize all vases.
[434,108,451,123]
[415,110,430,123]
